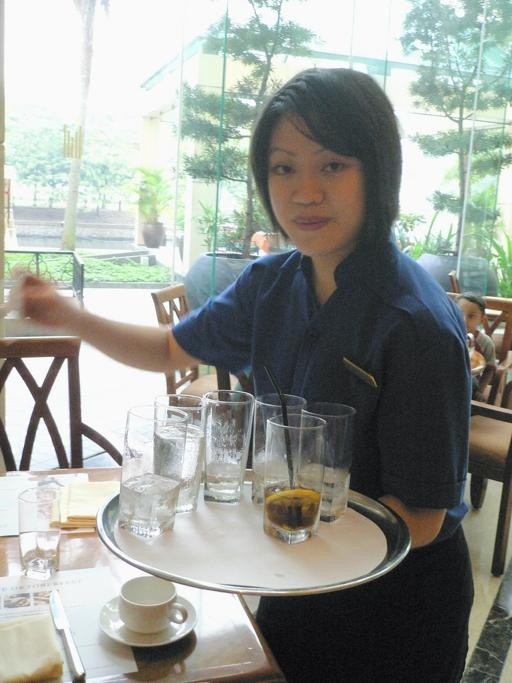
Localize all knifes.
[49,584,88,683]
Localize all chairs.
[469,401,512,576]
[447,291,512,406]
[152,285,246,450]
[448,270,506,360]
[0,336,122,471]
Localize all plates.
[98,594,197,647]
[96,467,412,596]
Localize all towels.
[51,480,123,529]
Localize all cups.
[152,392,209,514]
[297,402,356,525]
[117,405,189,540]
[252,392,307,506]
[264,413,327,544]
[117,575,188,634]
[202,389,255,503]
[18,488,61,578]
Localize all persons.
[2,64,476,682]
[455,291,497,401]
